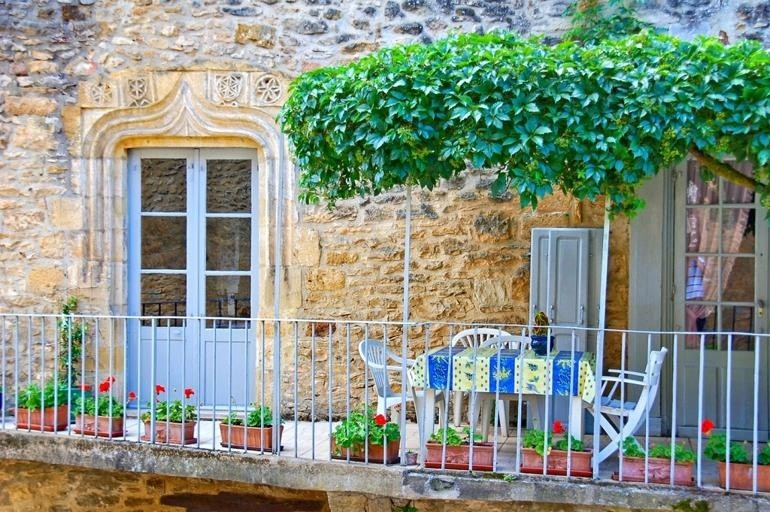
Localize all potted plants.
[424,427,493,471]
[10,384,68,431]
[614,437,695,485]
[219,392,285,452]
[531,312,555,355]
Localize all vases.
[521,446,592,476]
[329,435,401,465]
[72,412,128,436]
[719,460,770,494]
[141,422,197,444]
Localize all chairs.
[585,346,668,470]
[478,336,540,443]
[450,328,515,438]
[359,339,444,460]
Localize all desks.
[407,345,597,464]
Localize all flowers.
[139,382,198,423]
[522,420,582,456]
[700,421,770,467]
[74,376,135,416]
[332,405,401,455]
